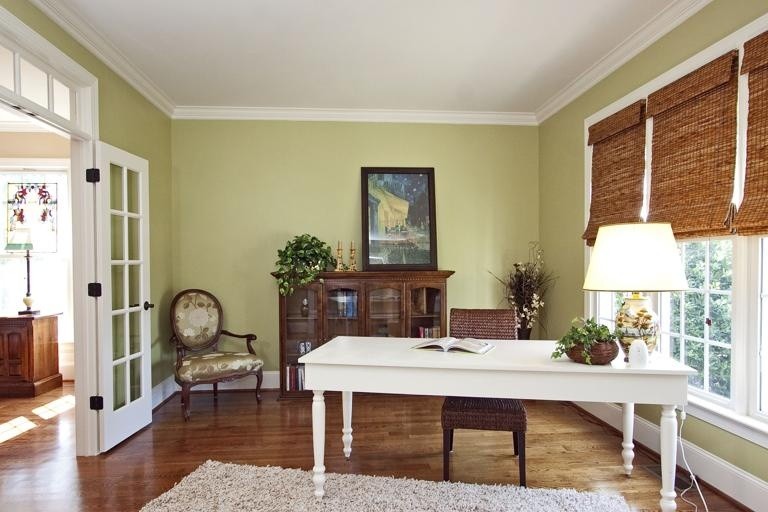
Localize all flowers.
[487,240,558,331]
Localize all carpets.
[136,459,631,512]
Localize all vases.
[516,327,531,340]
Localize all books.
[424,327,428,338]
[298,366,301,391]
[437,326,441,338]
[432,326,437,338]
[428,328,432,338]
[301,365,305,391]
[290,365,296,392]
[417,326,424,338]
[346,291,352,317]
[286,365,290,391]
[352,291,357,316]
[409,336,495,356]
[336,289,344,318]
[295,365,299,391]
[342,290,346,316]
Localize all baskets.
[565,340,619,365]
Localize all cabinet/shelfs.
[270,268,456,401]
[0,311,64,398]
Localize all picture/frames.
[359,165,438,271]
[7,181,58,254]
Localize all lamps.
[3,229,40,315]
[581,222,690,362]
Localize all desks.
[295,334,696,512]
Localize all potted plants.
[550,315,619,365]
[269,233,349,296]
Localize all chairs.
[440,307,527,487]
[166,288,264,419]
